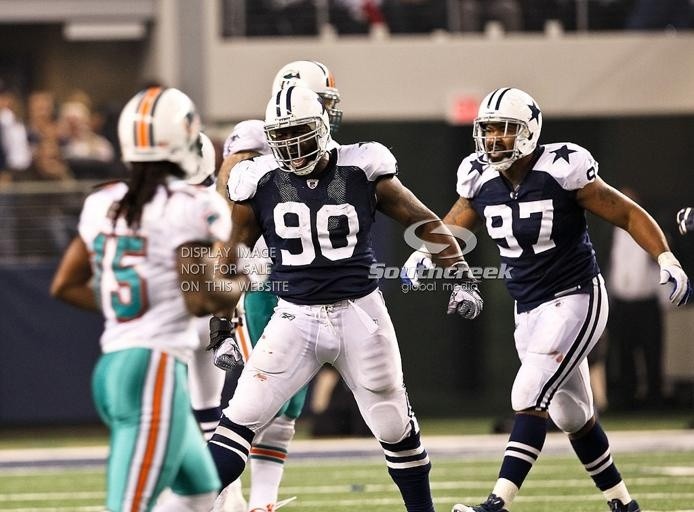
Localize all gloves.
[401,249,435,289]
[448,265,485,320]
[206,317,244,373]
[658,252,691,307]
[677,207,693,235]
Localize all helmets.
[263,61,342,174]
[473,88,543,173]
[117,86,217,186]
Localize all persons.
[676,205,694,235]
[591,179,667,416]
[156,131,248,512]
[47,84,270,512]
[205,84,438,512]
[400,86,690,512]
[243,0,694,41]
[0,81,164,183]
[205,60,490,512]
[217,116,268,512]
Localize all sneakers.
[453,494,511,512]
